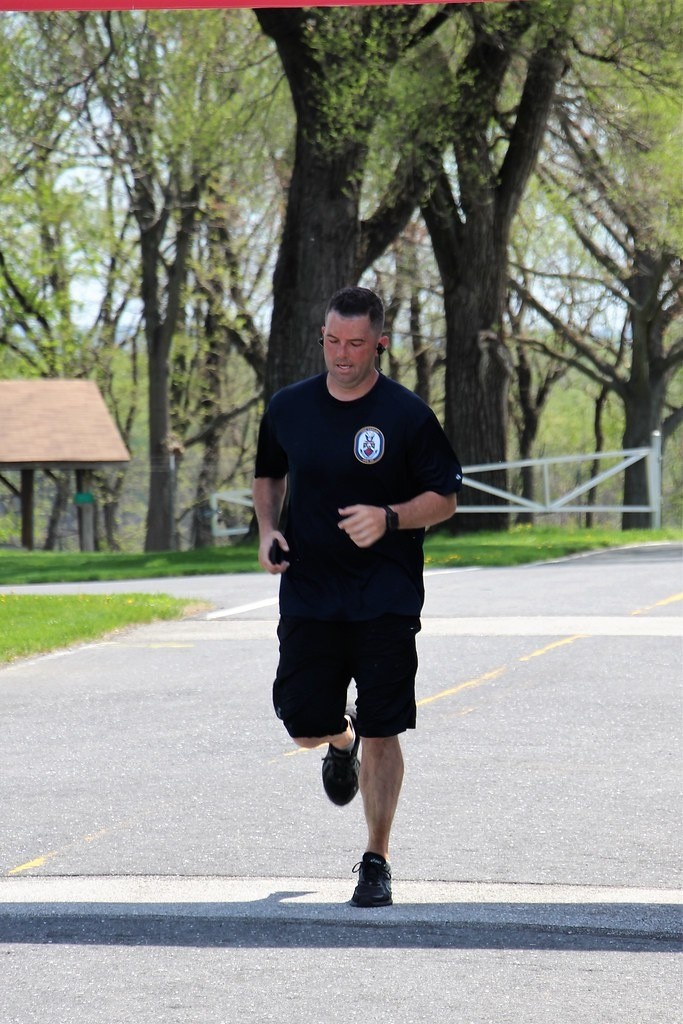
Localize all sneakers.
[350,852,393,908]
[321,708,361,806]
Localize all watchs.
[382,505,399,531]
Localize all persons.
[253,286,464,907]
